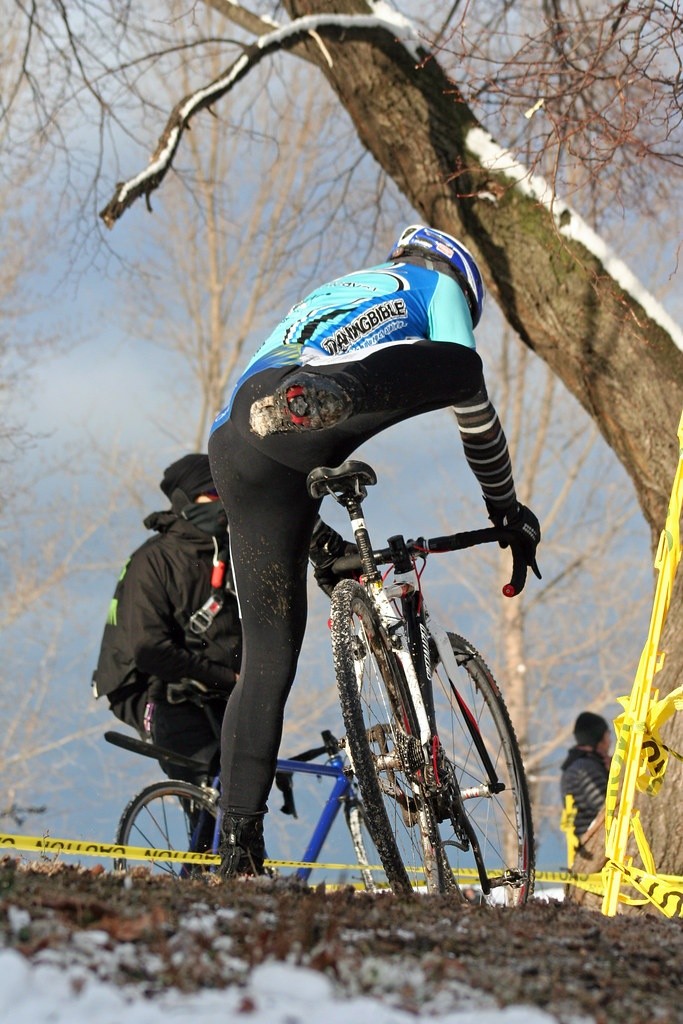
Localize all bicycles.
[309,459,533,906]
[102,726,489,897]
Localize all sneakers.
[245,373,364,436]
[221,812,273,876]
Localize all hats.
[575,711,610,747]
[161,454,219,502]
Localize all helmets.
[385,223,482,333]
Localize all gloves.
[489,502,540,554]
[306,527,362,599]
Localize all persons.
[91,453,244,875]
[559,712,612,840]
[208,223,541,877]
